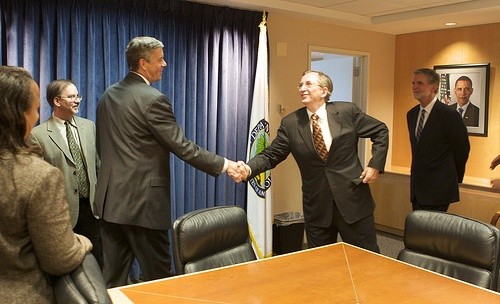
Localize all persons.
[28,80,104,271]
[237,70,389,254]
[0,64,92,304]
[449,76,479,127]
[93,36,246,289]
[406,69,470,212]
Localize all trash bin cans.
[273,211,305,254]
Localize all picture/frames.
[433,63,491,137]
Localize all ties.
[310,113,329,161]
[64,121,91,199]
[416,109,426,142]
[458,107,465,118]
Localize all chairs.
[172,205,257,274]
[398,210,500,291]
[53,249,111,304]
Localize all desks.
[107,242,500,304]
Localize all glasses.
[58,94,83,101]
[296,82,322,89]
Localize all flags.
[248,24,273,261]
